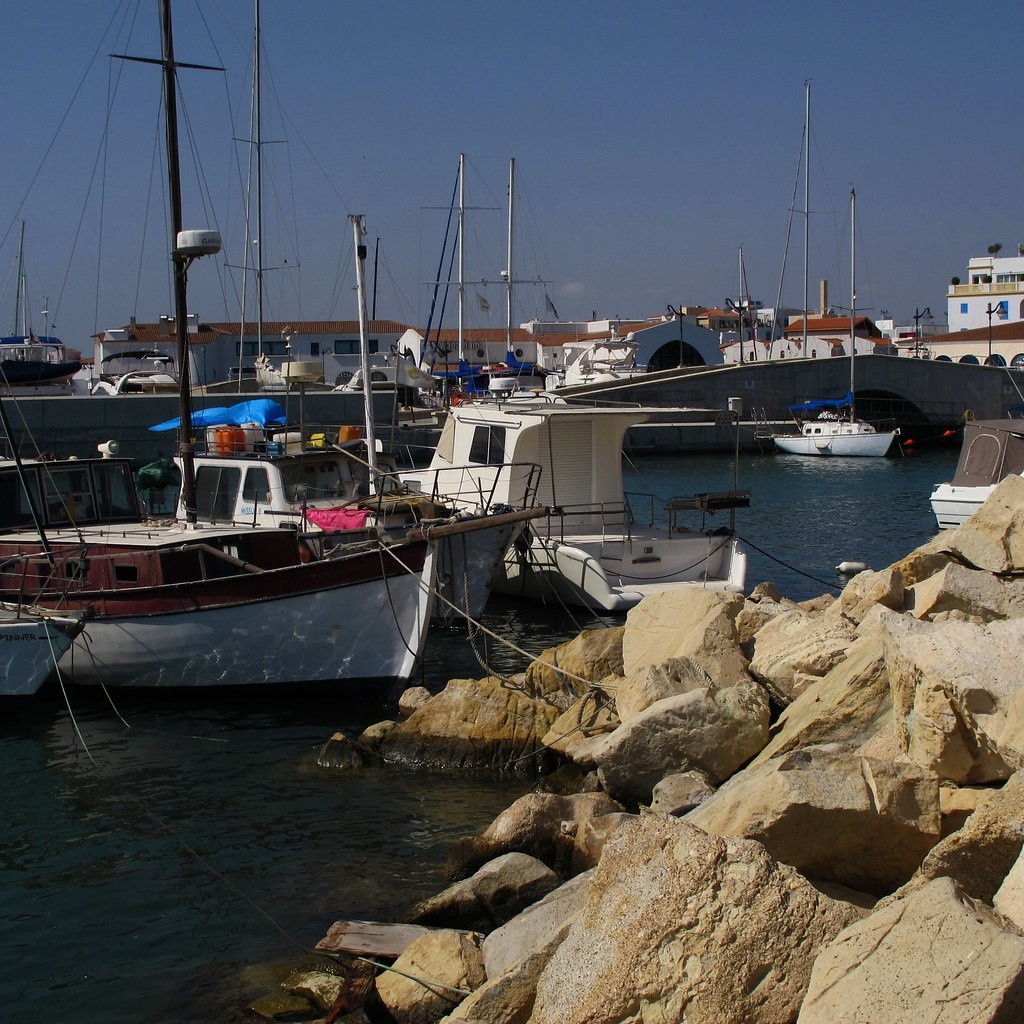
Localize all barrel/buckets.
[205,421,363,453]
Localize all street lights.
[913,308,933,358]
[986,301,1008,366]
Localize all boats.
[771,187,900,456]
[928,420,1024,529]
[1,0,749,695]
